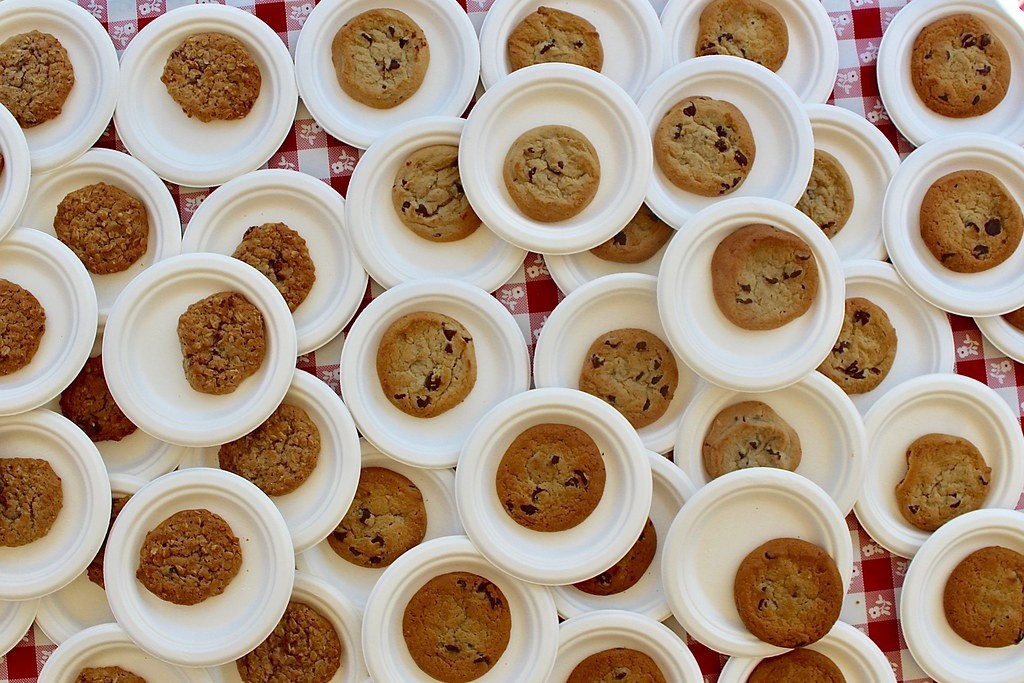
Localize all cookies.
[0,0,1024,683]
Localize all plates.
[0,0,1024,683]
